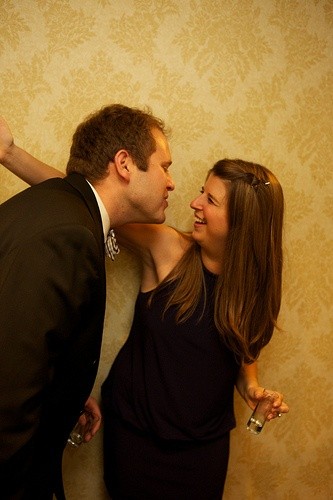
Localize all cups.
[68,411,100,448]
[246,387,279,434]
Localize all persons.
[0,114,292,500]
[0,104,176,499]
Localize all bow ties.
[105,228,121,260]
